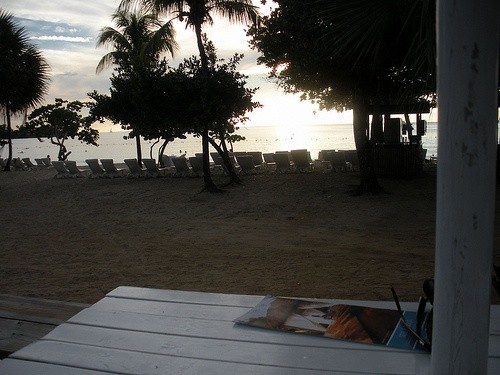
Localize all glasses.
[391,278,434,355]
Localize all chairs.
[0,150,357,178]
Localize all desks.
[0,286,500,375]
